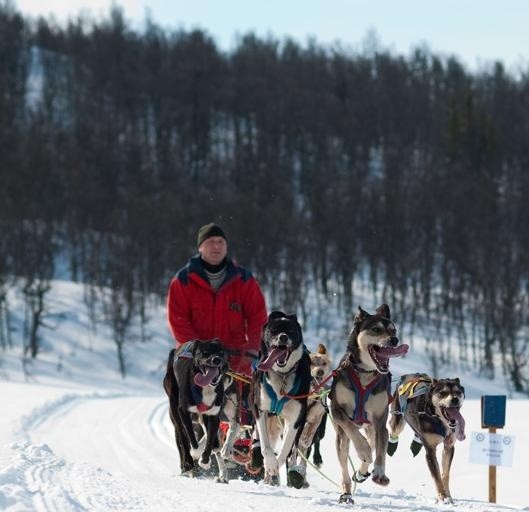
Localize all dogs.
[387,372,466,505]
[173,338,229,470]
[308,342,331,468]
[162,349,194,473]
[248,310,311,487]
[328,304,409,505]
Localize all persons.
[169,222,267,476]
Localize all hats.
[197,224,228,247]
